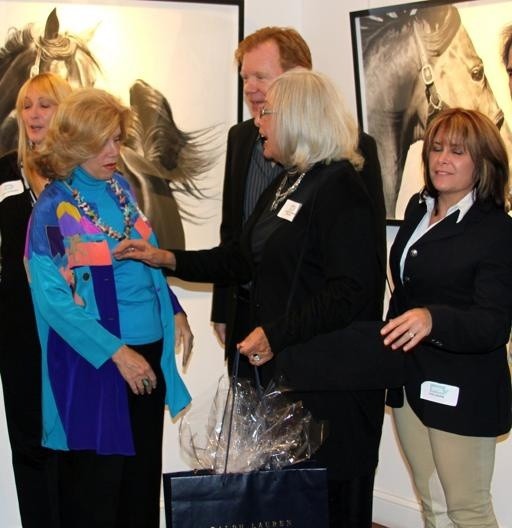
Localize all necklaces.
[269,169,310,212]
[63,174,132,242]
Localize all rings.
[407,330,414,338]
[252,354,260,362]
[143,377,151,386]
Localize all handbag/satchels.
[160,345,339,527]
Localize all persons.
[113,65,388,528]
[23,88,195,528]
[0,72,73,528]
[380,107,512,528]
[210,26,389,376]
[499,25,512,102]
